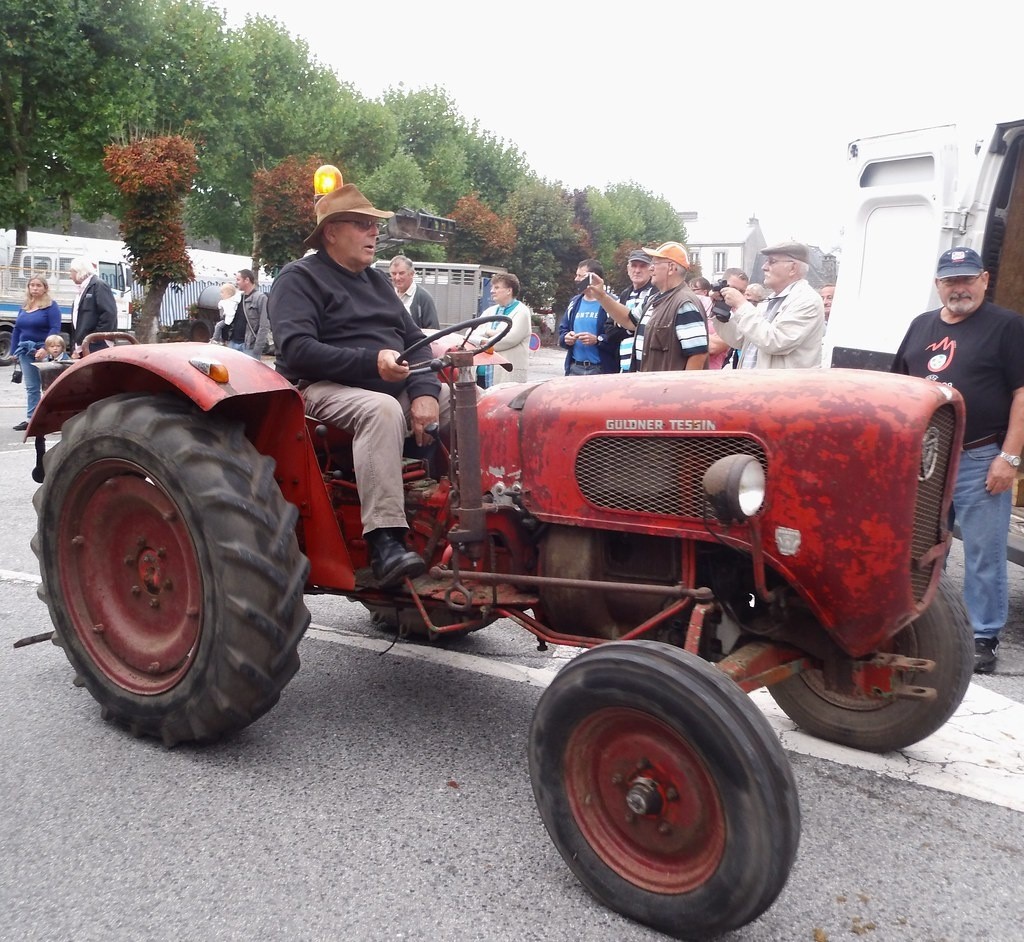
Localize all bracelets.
[594,335,604,346]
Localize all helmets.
[641,242,690,271]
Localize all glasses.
[651,261,672,266]
[691,289,700,292]
[326,219,380,232]
[766,259,793,265]
[939,274,981,288]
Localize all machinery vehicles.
[25,166,976,942]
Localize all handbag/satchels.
[263,338,275,356]
[11,362,22,383]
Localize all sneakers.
[973,636,1000,674]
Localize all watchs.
[998,451,1021,468]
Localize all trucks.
[0,244,133,366]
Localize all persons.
[266,184,484,591]
[9,273,61,431]
[708,241,825,369]
[228,269,270,361]
[68,256,118,360]
[388,255,440,330]
[586,241,709,373]
[208,283,242,347]
[557,241,837,376]
[889,247,1024,675]
[464,272,531,390]
[34,334,72,362]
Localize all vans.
[824,119,1024,566]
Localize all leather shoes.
[13,421,28,430]
[372,533,427,592]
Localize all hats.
[761,242,811,264]
[935,248,985,279]
[303,183,394,248]
[628,249,653,264]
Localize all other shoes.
[209,339,225,346]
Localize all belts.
[963,431,1003,450]
[572,359,600,369]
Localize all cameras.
[711,279,730,298]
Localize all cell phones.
[576,275,593,294]
